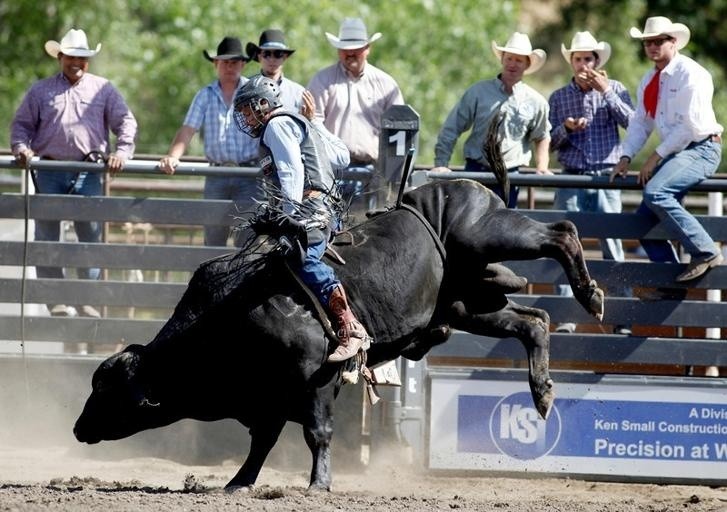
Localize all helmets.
[232,75,283,138]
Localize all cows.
[72,110,605,494]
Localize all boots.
[327,283,371,363]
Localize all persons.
[231,74,372,362]
[548,29,636,335]
[9,29,137,318]
[609,15,723,300]
[246,30,305,113]
[305,16,405,197]
[434,31,553,210]
[158,37,267,249]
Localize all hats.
[45,28,102,58]
[631,15,691,51]
[492,32,547,76]
[325,16,382,50]
[561,30,611,71]
[205,36,248,61]
[246,29,296,60]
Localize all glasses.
[641,36,673,48]
[260,49,285,60]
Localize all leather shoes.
[674,253,724,283]
[636,289,686,301]
